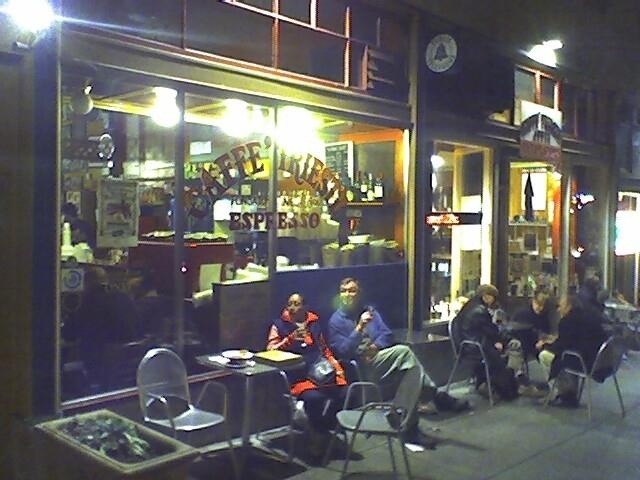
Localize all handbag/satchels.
[306,355,337,386]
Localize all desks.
[195,351,306,478]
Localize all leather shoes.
[548,394,579,408]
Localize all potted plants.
[34,409,201,480]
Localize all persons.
[534,294,603,408]
[327,276,470,449]
[457,282,525,400]
[60,204,95,249]
[504,284,560,398]
[67,220,92,245]
[267,292,348,459]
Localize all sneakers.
[403,426,437,449]
[433,392,469,412]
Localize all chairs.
[136,348,243,480]
[255,361,426,480]
[546,335,626,422]
[445,315,495,406]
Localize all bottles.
[346,171,386,202]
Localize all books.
[256,350,302,368]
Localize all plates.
[221,349,254,362]
